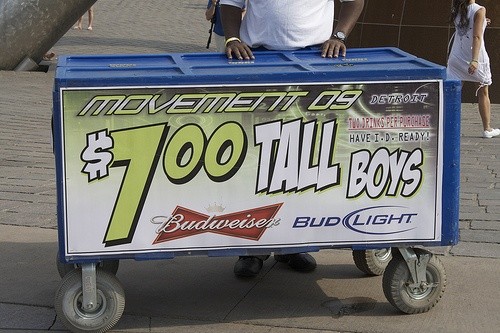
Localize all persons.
[446,0,500,138]
[206,0,246,52]
[73,7,93,30]
[221,0,364,279]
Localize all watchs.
[331,31,346,44]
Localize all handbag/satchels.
[205,0,218,19]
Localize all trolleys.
[51,46,462,333]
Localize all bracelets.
[225,37,241,47]
[472,59,478,63]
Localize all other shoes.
[74,26,81,29]
[88,27,92,30]
[233,255,264,278]
[274,252,316,272]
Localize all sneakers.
[483,128,500,138]
